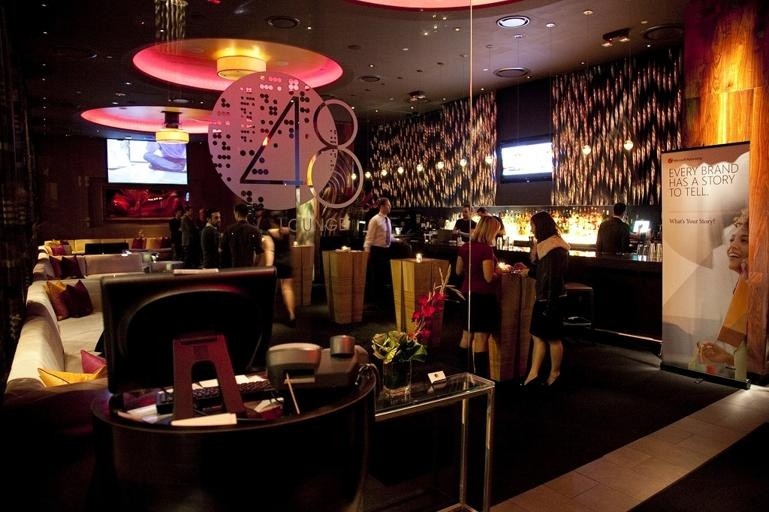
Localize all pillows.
[81,350,107,373]
[46,280,69,320]
[64,279,93,318]
[62,255,86,279]
[63,245,73,254]
[51,244,65,256]
[39,369,97,386]
[50,256,62,277]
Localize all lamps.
[156,112,189,144]
[217,56,266,81]
[601,27,630,47]
[409,90,426,102]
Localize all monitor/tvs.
[106,137,190,188]
[97,267,278,426]
[494,134,560,185]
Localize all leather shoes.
[287,318,295,326]
[517,376,538,387]
[542,373,562,387]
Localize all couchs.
[33,237,168,280]
[4,279,108,427]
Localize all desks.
[91,371,376,512]
[375,371,496,512]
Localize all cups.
[496,237,502,247]
[636,243,662,262]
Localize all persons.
[453,206,509,387]
[169,209,183,260]
[143,141,186,173]
[596,202,634,252]
[200,208,224,270]
[516,212,574,392]
[223,204,264,269]
[358,198,408,310]
[693,209,751,370]
[267,210,300,327]
[180,207,199,268]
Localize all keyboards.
[150,378,276,414]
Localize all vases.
[383,360,412,397]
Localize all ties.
[384,217,390,246]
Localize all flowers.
[371,263,466,365]
